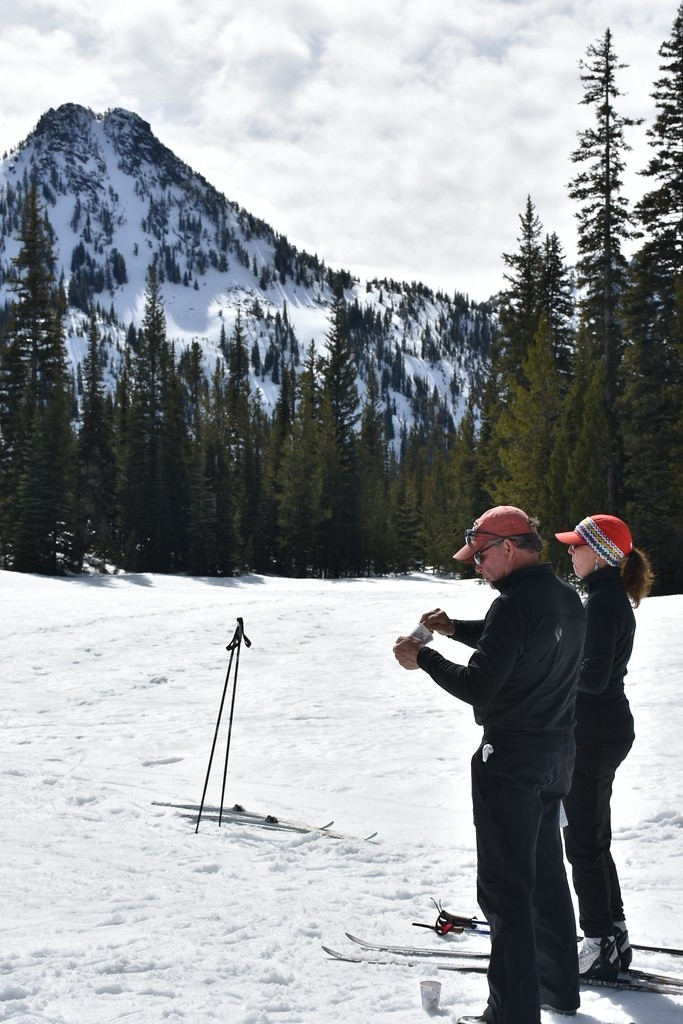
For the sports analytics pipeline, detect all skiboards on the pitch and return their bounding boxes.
[149,799,378,843]
[320,930,683,996]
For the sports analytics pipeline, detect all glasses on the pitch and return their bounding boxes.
[474,538,517,564]
[572,544,586,549]
[465,529,517,545]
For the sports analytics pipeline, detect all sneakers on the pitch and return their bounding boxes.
[613,921,632,969]
[577,934,621,979]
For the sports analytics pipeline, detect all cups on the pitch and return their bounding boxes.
[406,622,434,645]
[420,981,442,1009]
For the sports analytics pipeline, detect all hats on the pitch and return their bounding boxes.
[453,506,537,563]
[555,515,633,556]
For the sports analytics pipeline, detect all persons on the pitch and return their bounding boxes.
[393,506,587,1024]
[555,515,654,979]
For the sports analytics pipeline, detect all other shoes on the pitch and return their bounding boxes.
[540,1003,576,1016]
[457,1015,487,1024]
